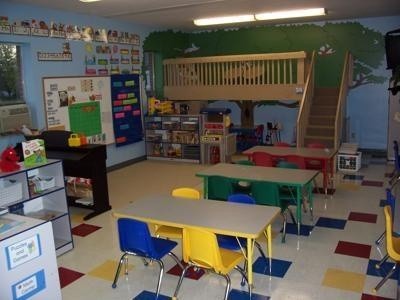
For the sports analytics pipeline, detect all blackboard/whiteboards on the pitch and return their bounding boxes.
[41,76,115,146]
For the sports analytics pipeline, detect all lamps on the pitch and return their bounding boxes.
[193,7,328,28]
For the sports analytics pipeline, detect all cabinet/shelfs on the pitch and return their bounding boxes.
[143,114,208,164]
[0,159,75,300]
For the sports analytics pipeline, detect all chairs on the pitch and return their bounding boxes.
[111,142,337,300]
[370,140,400,292]
